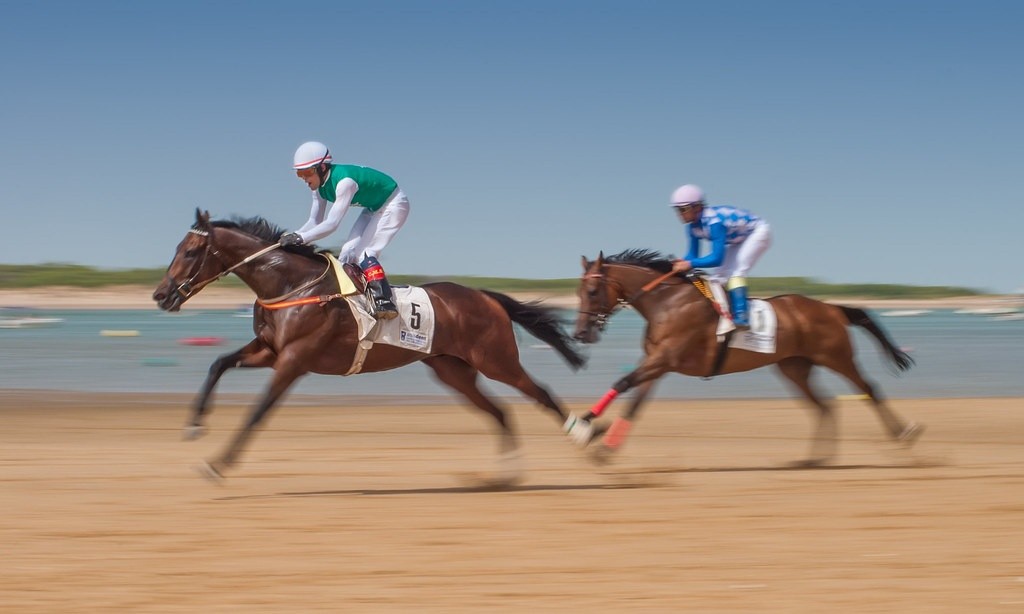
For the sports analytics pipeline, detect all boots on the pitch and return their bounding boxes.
[360,256,399,321]
[725,276,750,333]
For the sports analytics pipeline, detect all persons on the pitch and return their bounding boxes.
[670,184,772,329]
[277,141,411,320]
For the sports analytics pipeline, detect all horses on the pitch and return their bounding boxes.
[152,207,924,479]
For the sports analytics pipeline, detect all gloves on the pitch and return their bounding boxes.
[278,233,303,250]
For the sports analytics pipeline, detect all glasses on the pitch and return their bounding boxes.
[676,206,693,214]
[297,170,317,178]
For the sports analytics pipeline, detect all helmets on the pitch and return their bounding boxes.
[292,141,333,169]
[668,184,706,206]
